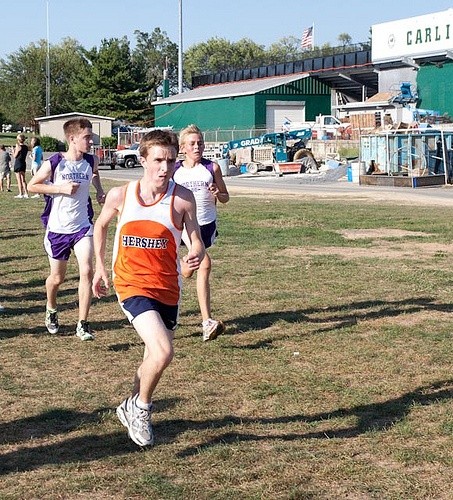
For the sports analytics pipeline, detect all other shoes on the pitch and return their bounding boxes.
[5,189,13,192]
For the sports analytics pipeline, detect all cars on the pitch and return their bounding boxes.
[113,142,143,168]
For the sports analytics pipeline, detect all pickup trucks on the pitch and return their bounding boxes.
[284,115,351,136]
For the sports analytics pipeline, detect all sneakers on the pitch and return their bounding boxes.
[116,393,154,446]
[76,321,94,340]
[202,318,225,342]
[45,305,58,334]
[14,194,29,198]
[31,194,41,198]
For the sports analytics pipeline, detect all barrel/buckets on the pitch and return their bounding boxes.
[351,161,366,184]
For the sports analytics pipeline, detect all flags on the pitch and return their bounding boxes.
[299,26,313,48]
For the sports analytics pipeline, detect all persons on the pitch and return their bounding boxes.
[169,123,229,342]
[28,136,43,199]
[92,129,206,447]
[0,133,28,198]
[27,117,106,340]
